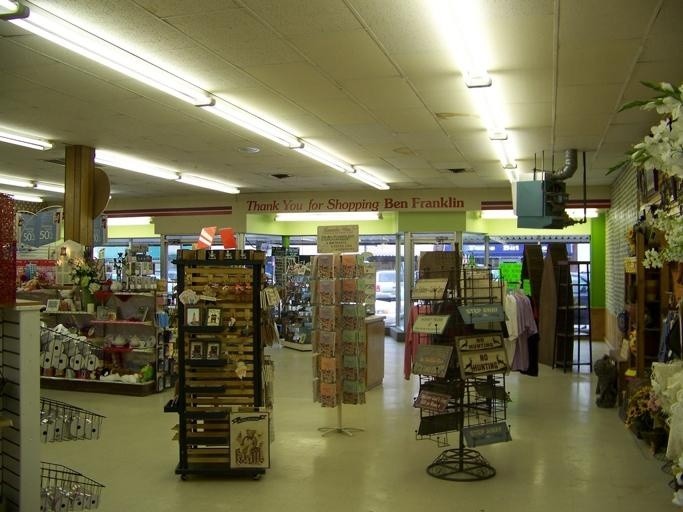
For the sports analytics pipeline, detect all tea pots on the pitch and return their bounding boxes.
[109,333,144,348]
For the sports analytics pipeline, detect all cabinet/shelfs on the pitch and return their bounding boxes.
[412,267,507,484]
[16,283,170,397]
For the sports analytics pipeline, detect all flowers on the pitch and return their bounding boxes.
[603,80,682,269]
[624,384,664,427]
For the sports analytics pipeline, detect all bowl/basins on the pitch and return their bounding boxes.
[60,289,74,298]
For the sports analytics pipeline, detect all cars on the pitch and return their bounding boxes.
[570,271,591,324]
[376,271,396,300]
